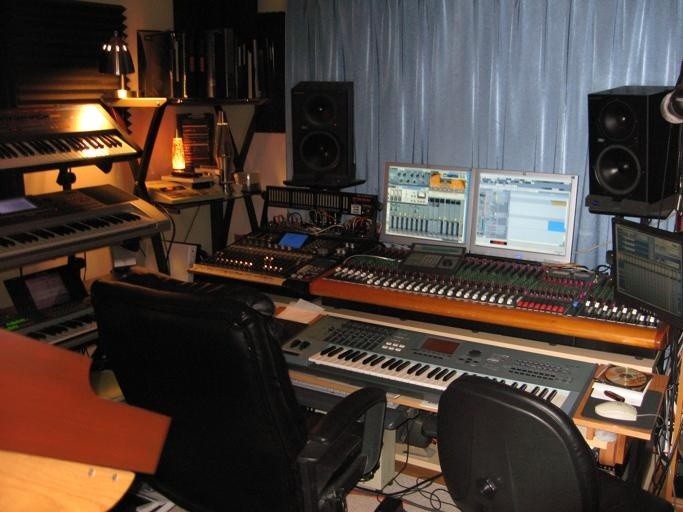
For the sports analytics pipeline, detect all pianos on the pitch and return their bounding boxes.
[0,286,99,351]
[279,313,598,425]
[0,102,145,175]
[0,184,172,271]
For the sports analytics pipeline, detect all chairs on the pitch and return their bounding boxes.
[90,272,387,512]
[436,375,683,512]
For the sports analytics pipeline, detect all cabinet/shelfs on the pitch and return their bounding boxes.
[101,97,262,275]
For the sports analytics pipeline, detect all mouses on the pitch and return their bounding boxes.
[594,401,637,422]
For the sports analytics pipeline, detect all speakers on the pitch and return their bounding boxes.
[588,86,683,220]
[290,81,356,188]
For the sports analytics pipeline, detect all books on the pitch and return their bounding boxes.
[175,112,214,170]
[133,27,266,100]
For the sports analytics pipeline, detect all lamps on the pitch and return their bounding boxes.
[96,30,135,99]
[170,129,193,177]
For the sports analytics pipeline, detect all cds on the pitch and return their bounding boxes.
[604,366,646,387]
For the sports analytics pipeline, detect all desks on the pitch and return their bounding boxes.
[189,241,683,512]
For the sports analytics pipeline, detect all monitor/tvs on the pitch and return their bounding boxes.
[470,168,579,268]
[612,217,683,331]
[381,162,475,254]
